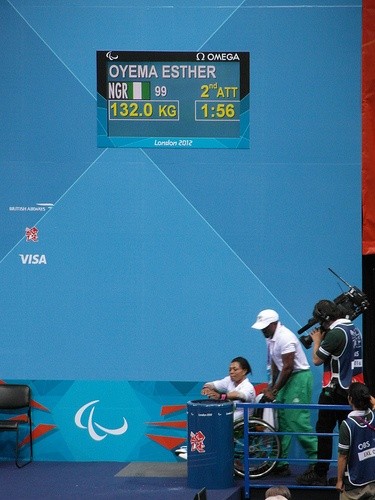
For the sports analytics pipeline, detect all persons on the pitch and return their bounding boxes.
[174,356,256,461]
[335,382,375,500]
[265,485,291,500]
[296,301,366,486]
[251,310,319,486]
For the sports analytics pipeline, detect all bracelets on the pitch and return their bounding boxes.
[202,386,210,389]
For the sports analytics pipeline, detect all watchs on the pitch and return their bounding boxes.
[270,386,279,396]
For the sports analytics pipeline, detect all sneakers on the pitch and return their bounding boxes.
[269,464,291,476]
[299,463,328,485]
[175,445,188,460]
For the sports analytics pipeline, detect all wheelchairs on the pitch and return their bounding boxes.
[232,392,283,480]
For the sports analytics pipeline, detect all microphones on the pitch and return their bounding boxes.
[298,319,317,334]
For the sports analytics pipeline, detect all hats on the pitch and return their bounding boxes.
[251,309,279,330]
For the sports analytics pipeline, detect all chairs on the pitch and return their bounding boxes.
[0,384,34,468]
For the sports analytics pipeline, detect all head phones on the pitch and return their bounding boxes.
[315,303,330,324]
